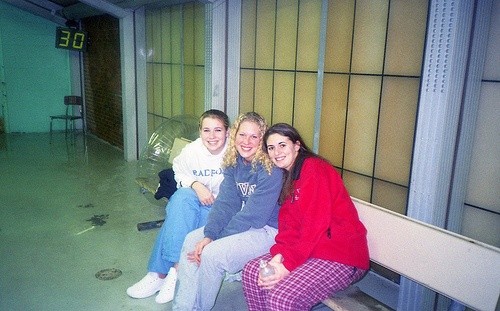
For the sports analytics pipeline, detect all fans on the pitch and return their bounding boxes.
[137,114,201,206]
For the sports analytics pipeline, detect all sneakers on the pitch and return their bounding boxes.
[156,267,177,304]
[126,272,166,298]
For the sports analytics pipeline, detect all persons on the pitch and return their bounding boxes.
[242,123,370,311]
[126,109,231,303]
[171,111,283,311]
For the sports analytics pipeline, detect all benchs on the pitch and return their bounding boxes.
[134,137,500,311]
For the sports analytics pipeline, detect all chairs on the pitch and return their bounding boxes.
[49,96,86,136]
